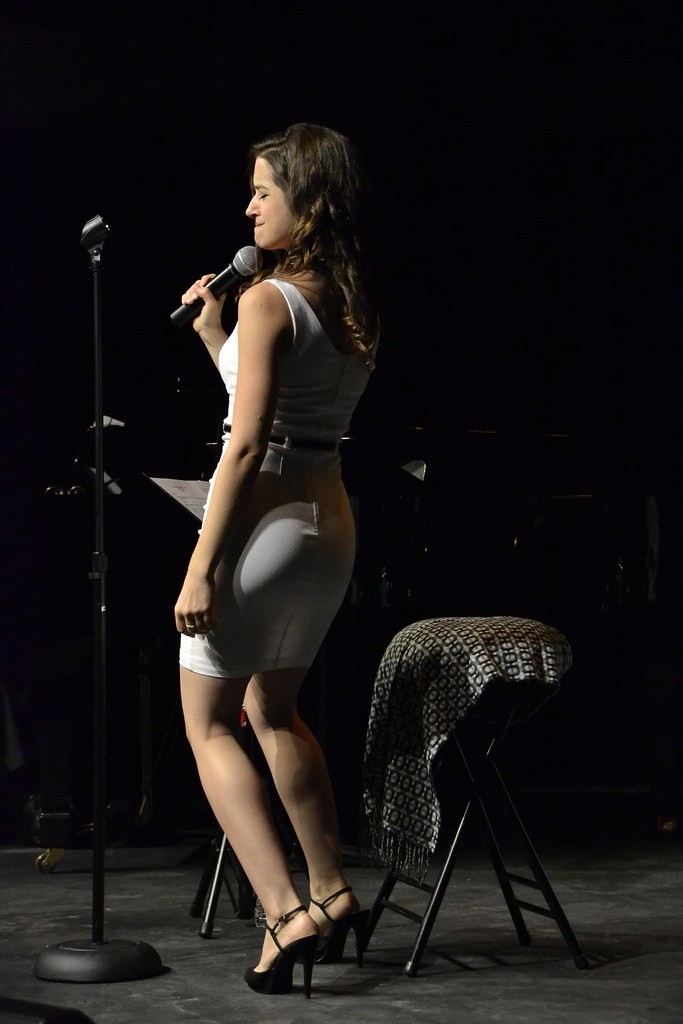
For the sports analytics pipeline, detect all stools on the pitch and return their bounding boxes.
[360,615,590,978]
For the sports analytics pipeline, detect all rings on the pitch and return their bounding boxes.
[186,624,194,630]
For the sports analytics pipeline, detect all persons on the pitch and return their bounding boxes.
[174,123,382,998]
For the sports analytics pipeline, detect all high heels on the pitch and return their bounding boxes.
[295,883,374,972]
[243,905,320,999]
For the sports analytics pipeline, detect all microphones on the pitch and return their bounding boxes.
[170,246,264,327]
